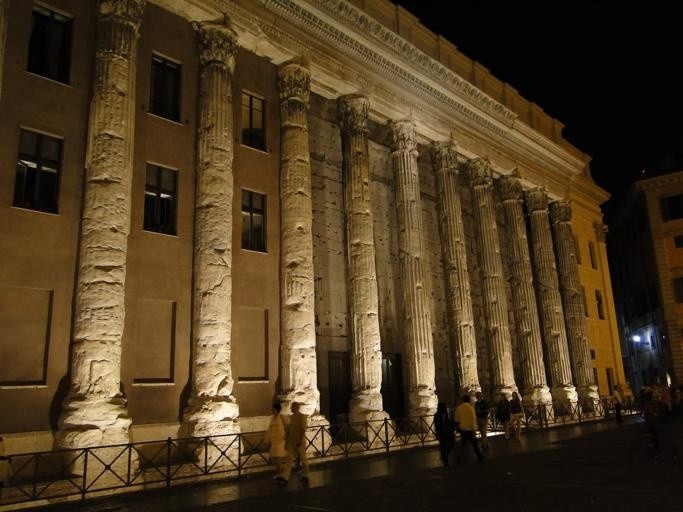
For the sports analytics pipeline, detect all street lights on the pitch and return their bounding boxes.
[624,325,640,416]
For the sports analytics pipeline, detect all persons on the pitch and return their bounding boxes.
[434,402,460,471]
[279,402,309,481]
[613,385,624,424]
[452,395,491,472]
[266,403,292,483]
[474,392,489,436]
[496,394,512,440]
[510,391,525,439]
